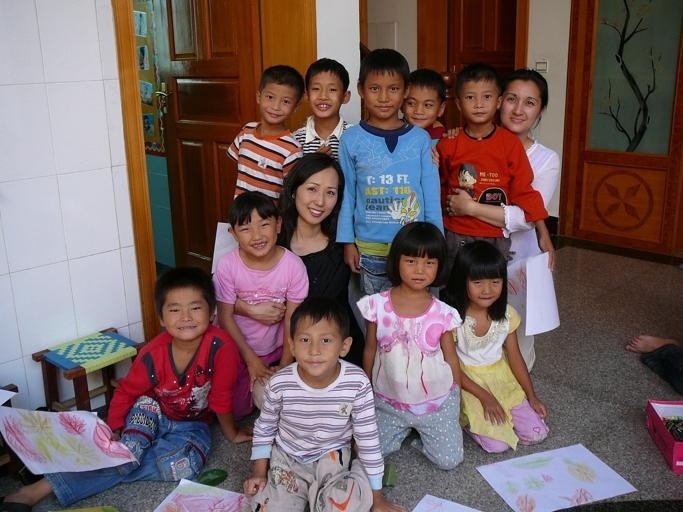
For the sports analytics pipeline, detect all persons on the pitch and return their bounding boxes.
[624,335,683,396]
[0,266,255,512]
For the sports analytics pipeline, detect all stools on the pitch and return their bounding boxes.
[0,383,23,478]
[32,327,145,414]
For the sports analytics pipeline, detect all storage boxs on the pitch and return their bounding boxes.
[646,399,683,474]
